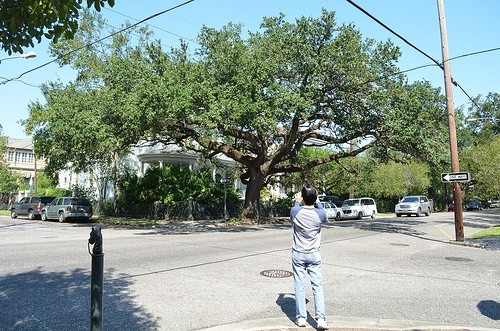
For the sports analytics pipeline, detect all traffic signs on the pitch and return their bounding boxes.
[441,172,472,183]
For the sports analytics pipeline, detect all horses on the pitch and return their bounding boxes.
[88,226,102,254]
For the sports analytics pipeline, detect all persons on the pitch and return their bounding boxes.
[289,185,329,330]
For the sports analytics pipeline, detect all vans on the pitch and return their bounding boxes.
[339,197,377,220]
[319,196,341,206]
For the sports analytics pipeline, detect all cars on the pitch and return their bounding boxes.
[490,202,500,208]
[448,200,483,212]
[320,202,343,221]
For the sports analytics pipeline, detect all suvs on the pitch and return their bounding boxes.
[39,197,92,223]
[9,196,57,220]
[394,196,431,217]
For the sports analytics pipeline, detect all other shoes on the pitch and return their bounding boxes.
[315,318,328,329]
[295,317,307,327]
